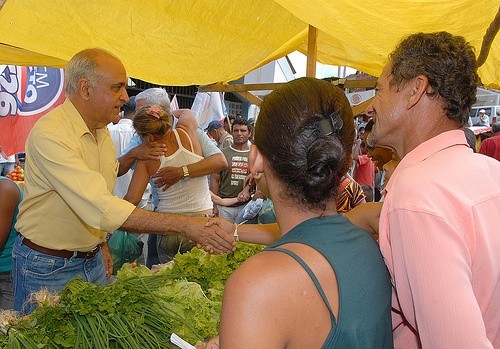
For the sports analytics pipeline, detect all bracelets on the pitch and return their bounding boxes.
[233,220,248,243]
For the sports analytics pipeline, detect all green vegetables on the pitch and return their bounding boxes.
[9,276,149,349]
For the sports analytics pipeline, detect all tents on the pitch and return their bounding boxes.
[1,0,499,121]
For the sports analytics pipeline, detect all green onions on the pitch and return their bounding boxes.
[0,274,204,349]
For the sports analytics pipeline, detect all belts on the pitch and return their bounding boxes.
[19,233,102,259]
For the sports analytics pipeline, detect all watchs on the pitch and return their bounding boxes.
[181,165,191,182]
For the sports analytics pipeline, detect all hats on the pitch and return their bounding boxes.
[479,109,485,113]
[204,120,221,132]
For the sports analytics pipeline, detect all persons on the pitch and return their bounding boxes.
[336,176,366,215]
[203,115,267,225]
[196,149,401,255]
[352,114,394,203]
[195,76,394,349]
[115,87,228,271]
[366,31,500,349]
[11,48,237,320]
[464,108,500,162]
[0,175,25,312]
[0,147,20,178]
[120,103,214,264]
[107,96,155,267]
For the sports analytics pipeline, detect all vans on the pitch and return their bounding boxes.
[471,105,500,126]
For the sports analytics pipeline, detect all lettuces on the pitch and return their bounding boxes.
[114,242,270,344]
